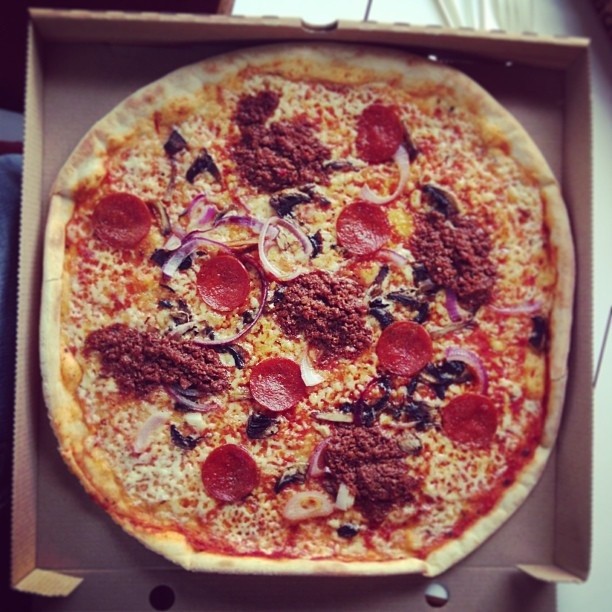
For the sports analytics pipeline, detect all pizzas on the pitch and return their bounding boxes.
[38,37,578,579]
